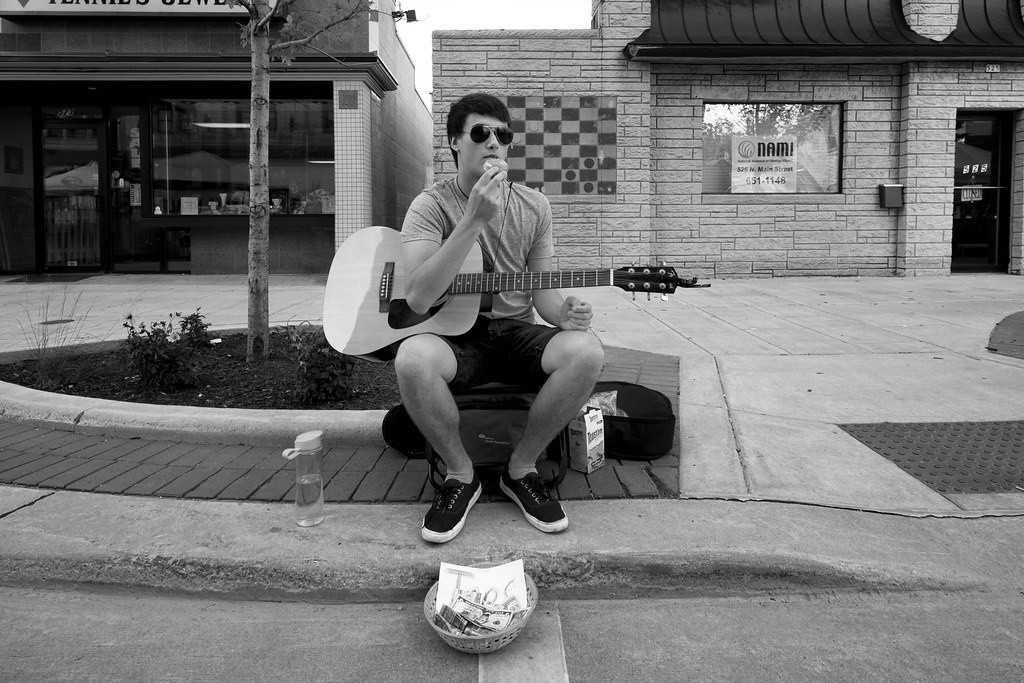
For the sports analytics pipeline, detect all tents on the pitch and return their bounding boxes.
[44,160,99,196]
[153,150,290,214]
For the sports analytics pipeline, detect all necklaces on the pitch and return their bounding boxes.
[456,175,469,198]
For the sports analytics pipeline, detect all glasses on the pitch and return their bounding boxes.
[461,122,515,147]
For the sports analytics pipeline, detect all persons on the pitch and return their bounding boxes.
[395,93,604,542]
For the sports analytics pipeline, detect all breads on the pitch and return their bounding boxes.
[483,158,508,177]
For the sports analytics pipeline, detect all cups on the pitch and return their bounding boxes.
[282,431,324,527]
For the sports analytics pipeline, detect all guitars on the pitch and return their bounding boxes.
[323,225,714,364]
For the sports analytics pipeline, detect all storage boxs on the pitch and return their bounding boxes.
[879,183,904,208]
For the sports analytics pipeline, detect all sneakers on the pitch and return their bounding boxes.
[497,465,570,533]
[419,467,482,544]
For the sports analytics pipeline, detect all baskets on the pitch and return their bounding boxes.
[423,561,539,653]
